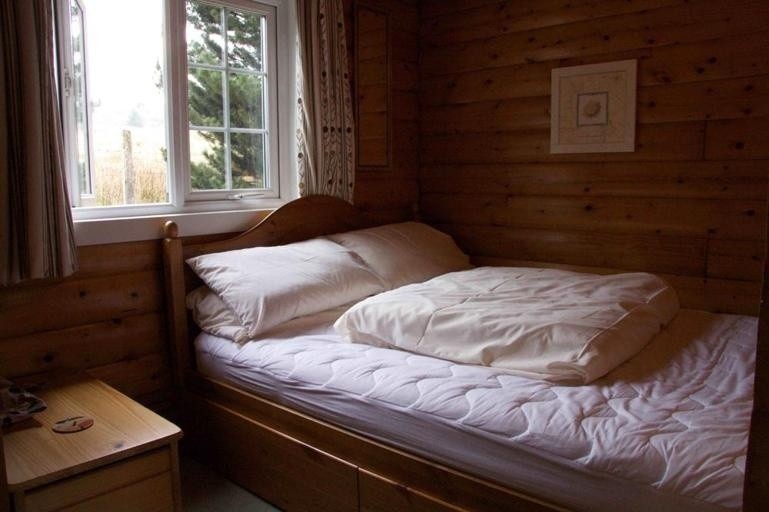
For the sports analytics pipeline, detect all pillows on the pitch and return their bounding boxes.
[332,220,475,289]
[185,236,385,338]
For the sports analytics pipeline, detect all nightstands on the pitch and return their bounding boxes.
[0,376,182,512]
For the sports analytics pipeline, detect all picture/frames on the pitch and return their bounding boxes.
[550,59,638,155]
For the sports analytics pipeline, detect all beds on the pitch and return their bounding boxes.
[161,193,759,512]
